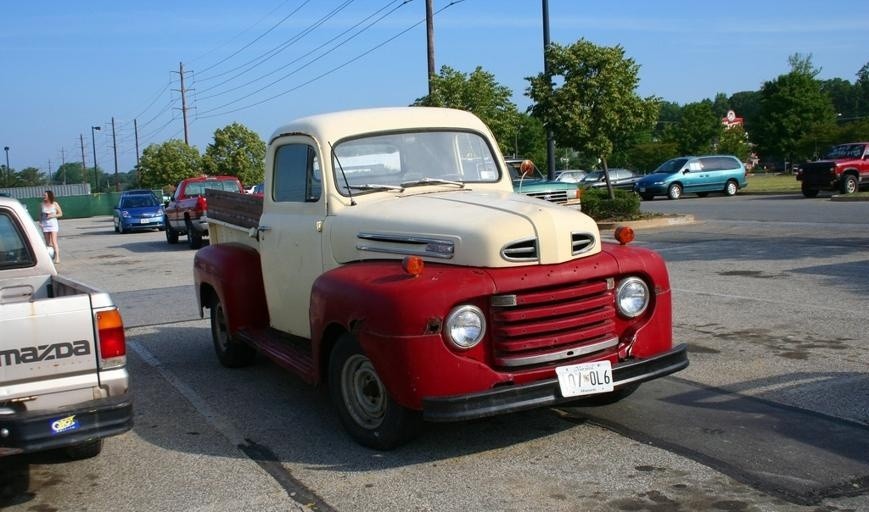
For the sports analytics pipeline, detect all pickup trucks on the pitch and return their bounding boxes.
[0,195,136,460]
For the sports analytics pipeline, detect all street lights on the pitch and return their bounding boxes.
[4,145,11,187]
[91,126,103,192]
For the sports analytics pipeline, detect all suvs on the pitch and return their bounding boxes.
[796,141,869,199]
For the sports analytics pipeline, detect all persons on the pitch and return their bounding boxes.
[37,189,63,264]
